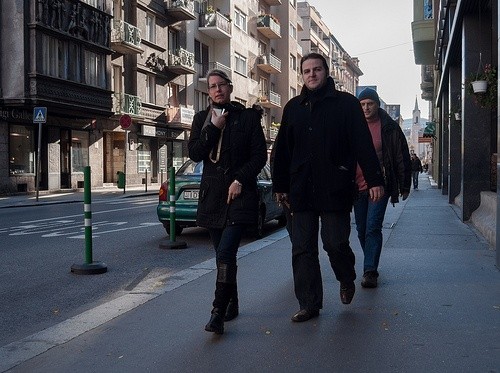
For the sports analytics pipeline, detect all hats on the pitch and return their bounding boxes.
[358,88,380,105]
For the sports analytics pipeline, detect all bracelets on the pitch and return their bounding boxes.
[234,180,243,185]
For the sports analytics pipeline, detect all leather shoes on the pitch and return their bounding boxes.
[292,309,320,322]
[339,280,355,305]
[361,277,377,287]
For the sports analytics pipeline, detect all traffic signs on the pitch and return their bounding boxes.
[33,107,47,123]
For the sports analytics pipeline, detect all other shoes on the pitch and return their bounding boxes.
[416,187,418,190]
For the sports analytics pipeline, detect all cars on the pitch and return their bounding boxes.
[157,159,286,237]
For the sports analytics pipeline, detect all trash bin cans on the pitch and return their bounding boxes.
[117,171,124,188]
[142,178,146,184]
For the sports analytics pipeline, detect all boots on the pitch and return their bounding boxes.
[205,263,238,335]
[211,266,238,320]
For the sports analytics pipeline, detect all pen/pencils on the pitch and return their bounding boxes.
[222,108,225,115]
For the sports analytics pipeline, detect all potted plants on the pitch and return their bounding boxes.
[465,64,498,111]
[448,105,463,121]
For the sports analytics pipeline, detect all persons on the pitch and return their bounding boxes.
[267,52,385,322]
[410,154,424,190]
[420,162,428,174]
[189,69,269,337]
[344,86,412,289]
